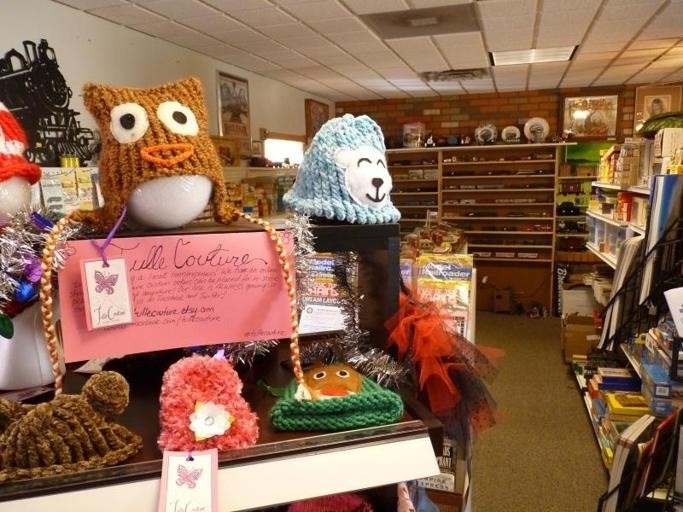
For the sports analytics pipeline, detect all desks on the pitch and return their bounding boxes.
[0,344,441,512]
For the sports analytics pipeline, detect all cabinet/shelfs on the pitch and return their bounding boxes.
[218,161,300,230]
[583,181,683,510]
[387,148,440,242]
[553,144,613,266]
[441,143,553,318]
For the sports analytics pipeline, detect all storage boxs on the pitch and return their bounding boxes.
[561,317,605,365]
[561,283,601,320]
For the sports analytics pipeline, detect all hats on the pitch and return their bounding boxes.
[283,113,402,224]
[269,359,405,433]
[0,102,41,187]
[72,76,239,230]
[0,370,144,485]
[156,355,260,454]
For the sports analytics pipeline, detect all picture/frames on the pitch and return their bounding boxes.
[215,68,251,144]
[555,86,682,144]
